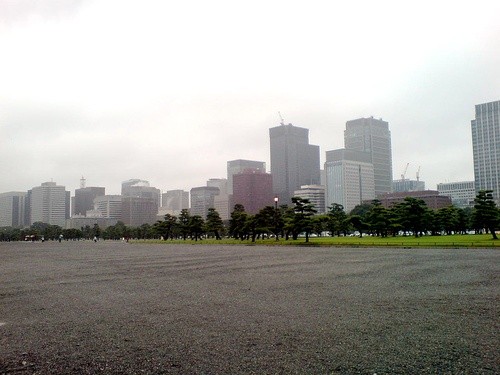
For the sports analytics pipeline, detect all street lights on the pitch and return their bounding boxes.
[274,192,279,242]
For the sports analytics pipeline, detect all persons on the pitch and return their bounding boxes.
[21,232,127,243]
